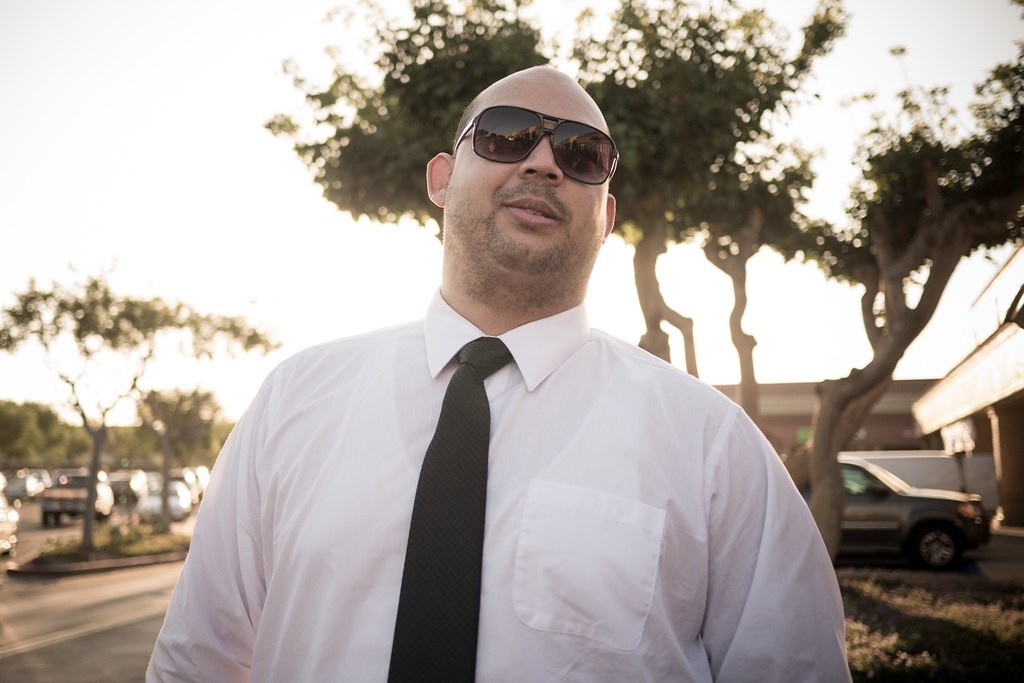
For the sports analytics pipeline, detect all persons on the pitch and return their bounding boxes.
[146,66,850,682]
[484,140,500,161]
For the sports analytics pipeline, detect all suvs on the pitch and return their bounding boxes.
[834,453,993,574]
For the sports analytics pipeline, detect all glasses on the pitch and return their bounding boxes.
[451,105,619,185]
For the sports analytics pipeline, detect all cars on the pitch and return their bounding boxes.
[0,467,210,559]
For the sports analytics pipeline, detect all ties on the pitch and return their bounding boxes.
[388,335,513,683]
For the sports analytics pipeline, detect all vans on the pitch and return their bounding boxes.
[836,445,1000,519]
